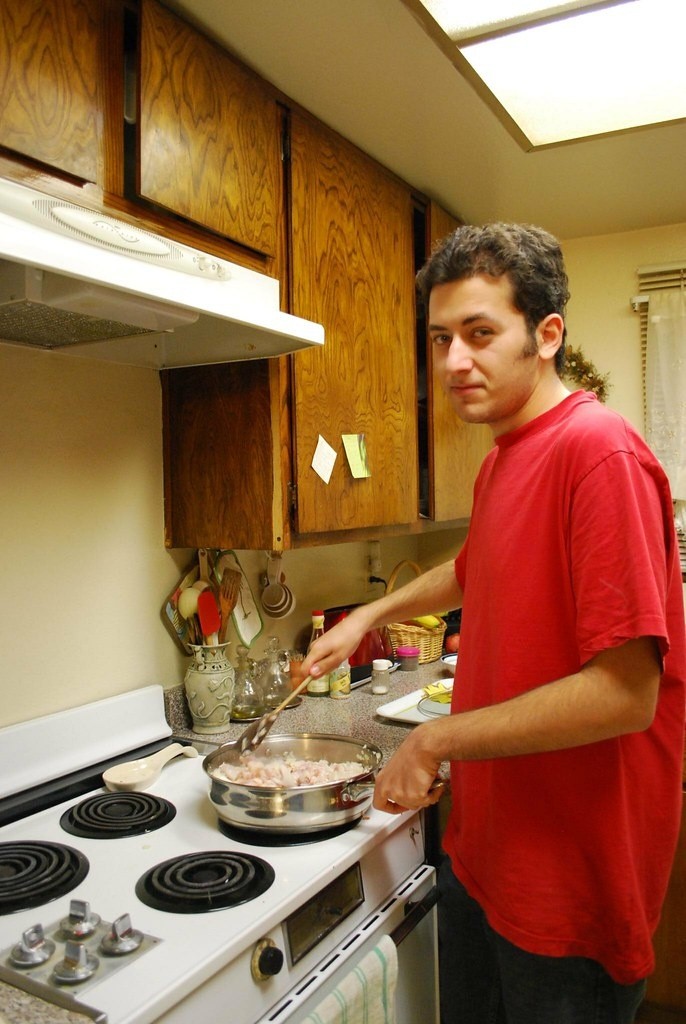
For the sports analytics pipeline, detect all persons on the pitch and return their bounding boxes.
[298,220,682,1024]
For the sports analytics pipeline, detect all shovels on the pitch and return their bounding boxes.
[234,676,316,755]
[218,569,243,645]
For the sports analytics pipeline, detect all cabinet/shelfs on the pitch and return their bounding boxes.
[141,812,440,1024]
[160,98,495,556]
[1,1,286,276]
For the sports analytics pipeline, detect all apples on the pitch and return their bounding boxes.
[445,632,460,653]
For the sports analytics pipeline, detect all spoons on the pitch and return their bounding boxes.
[261,555,296,620]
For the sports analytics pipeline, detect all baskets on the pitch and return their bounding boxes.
[382,560,448,664]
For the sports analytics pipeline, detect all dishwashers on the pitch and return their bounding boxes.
[252,864,442,1024]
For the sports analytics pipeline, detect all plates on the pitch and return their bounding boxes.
[376,678,454,725]
[230,708,274,722]
[266,696,304,710]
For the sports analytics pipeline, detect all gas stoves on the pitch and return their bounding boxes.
[1,683,430,1024]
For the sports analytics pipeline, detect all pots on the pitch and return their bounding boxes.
[202,732,384,835]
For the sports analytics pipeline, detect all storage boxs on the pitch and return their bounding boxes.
[384,560,447,665]
[184,639,237,735]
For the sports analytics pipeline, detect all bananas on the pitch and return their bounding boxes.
[409,612,448,628]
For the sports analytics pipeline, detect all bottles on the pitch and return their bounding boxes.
[329,612,351,699]
[230,645,264,719]
[258,636,293,707]
[306,610,329,697]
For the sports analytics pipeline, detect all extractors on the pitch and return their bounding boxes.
[0,174,326,372]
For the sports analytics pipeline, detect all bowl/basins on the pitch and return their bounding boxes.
[441,652,458,675]
